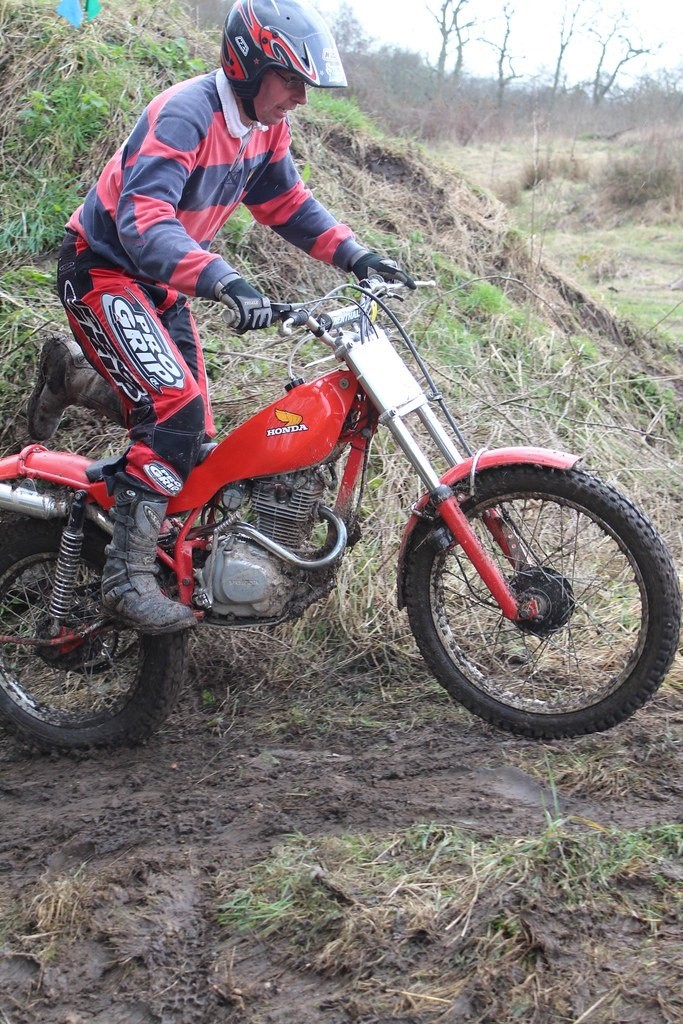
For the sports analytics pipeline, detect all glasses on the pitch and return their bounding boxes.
[271,67,307,89]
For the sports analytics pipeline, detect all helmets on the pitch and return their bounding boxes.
[220,0,349,99]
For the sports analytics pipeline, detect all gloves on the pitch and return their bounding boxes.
[352,253,417,289]
[219,278,272,334]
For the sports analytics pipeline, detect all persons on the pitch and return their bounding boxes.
[28,0,416,632]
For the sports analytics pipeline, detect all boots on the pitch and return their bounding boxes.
[103,483,198,636]
[25,338,128,442]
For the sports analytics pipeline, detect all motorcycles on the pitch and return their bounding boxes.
[0,274,682,752]
